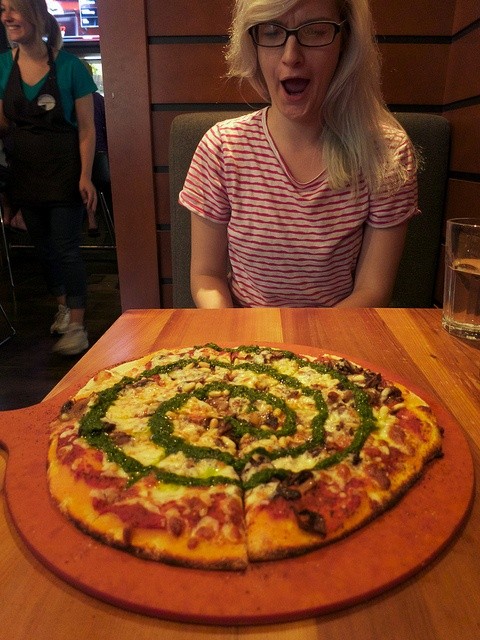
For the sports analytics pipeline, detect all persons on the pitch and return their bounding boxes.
[0,0,95,355]
[177,1,420,308]
[80,57,111,187]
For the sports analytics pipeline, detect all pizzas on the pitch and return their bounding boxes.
[46,342,441,570]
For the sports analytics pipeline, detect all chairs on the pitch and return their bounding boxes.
[170,112,452,309]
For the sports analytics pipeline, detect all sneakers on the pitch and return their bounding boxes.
[50,304,71,335]
[53,322,89,355]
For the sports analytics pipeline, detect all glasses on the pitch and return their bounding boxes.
[248,17,348,48]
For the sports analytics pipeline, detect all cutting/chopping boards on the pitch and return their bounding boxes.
[0,342,478,622]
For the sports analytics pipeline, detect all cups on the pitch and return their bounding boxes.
[443,217,480,342]
